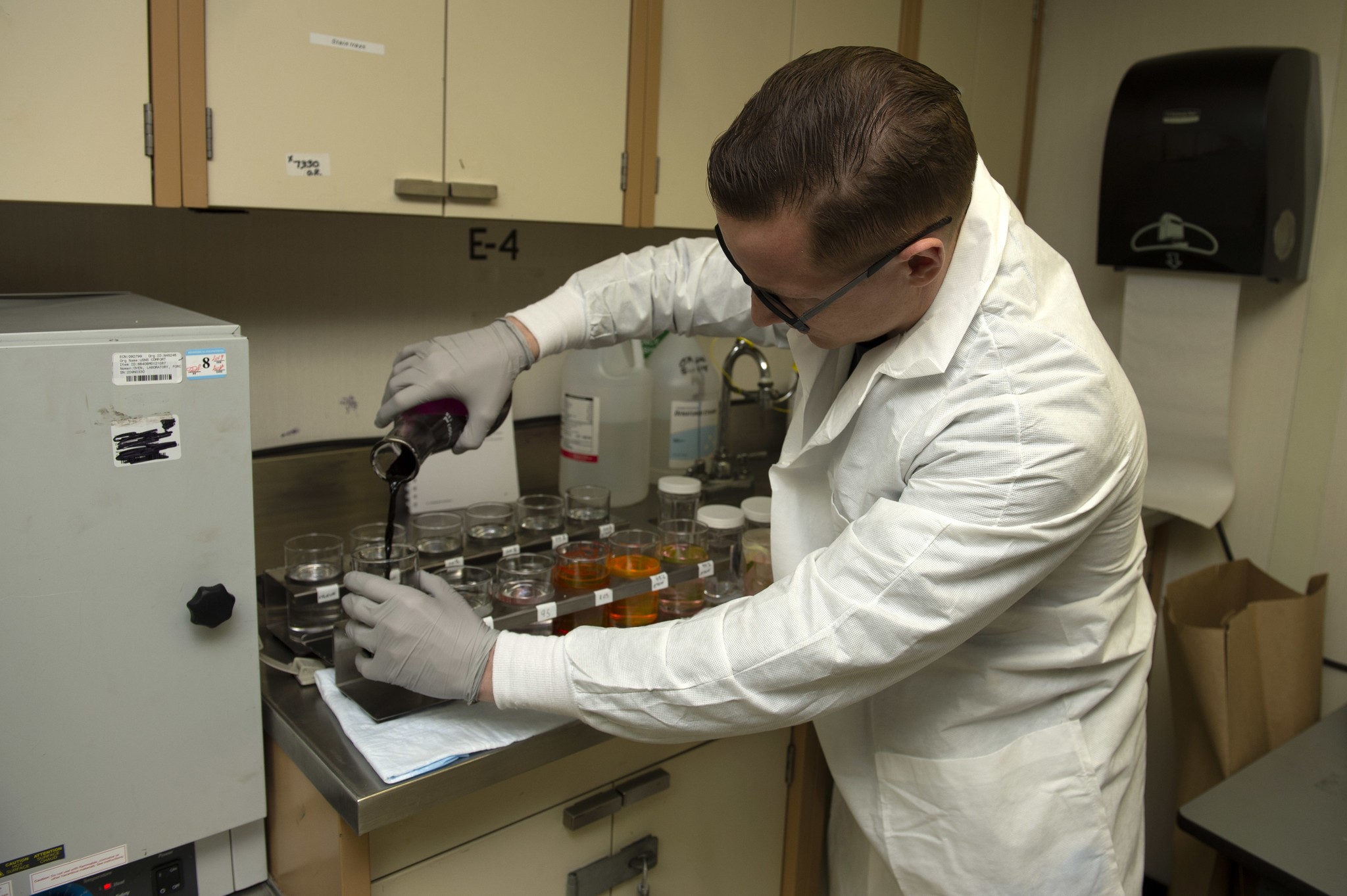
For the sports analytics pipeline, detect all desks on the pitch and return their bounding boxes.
[1176,704,1347,896]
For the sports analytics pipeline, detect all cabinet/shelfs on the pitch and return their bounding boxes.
[0,0,1048,232]
[262,723,833,896]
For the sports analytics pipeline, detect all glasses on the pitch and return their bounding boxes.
[714,214,951,334]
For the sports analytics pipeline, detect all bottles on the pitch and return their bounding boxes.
[647,332,721,482]
[559,337,652,508]
[370,390,513,484]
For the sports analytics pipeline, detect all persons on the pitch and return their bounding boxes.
[342,45,1157,896]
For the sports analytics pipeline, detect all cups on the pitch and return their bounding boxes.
[566,485,611,528]
[658,519,709,614]
[494,552,557,636]
[517,494,565,536]
[556,541,611,633]
[741,497,774,596]
[351,543,420,590]
[283,534,345,643]
[349,523,408,571]
[657,476,702,544]
[609,529,662,628]
[696,504,744,604]
[433,565,493,611]
[464,501,519,546]
[410,511,466,573]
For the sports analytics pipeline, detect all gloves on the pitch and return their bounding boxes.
[374,318,535,455]
[343,569,500,706]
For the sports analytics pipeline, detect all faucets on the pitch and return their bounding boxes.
[685,341,776,490]
